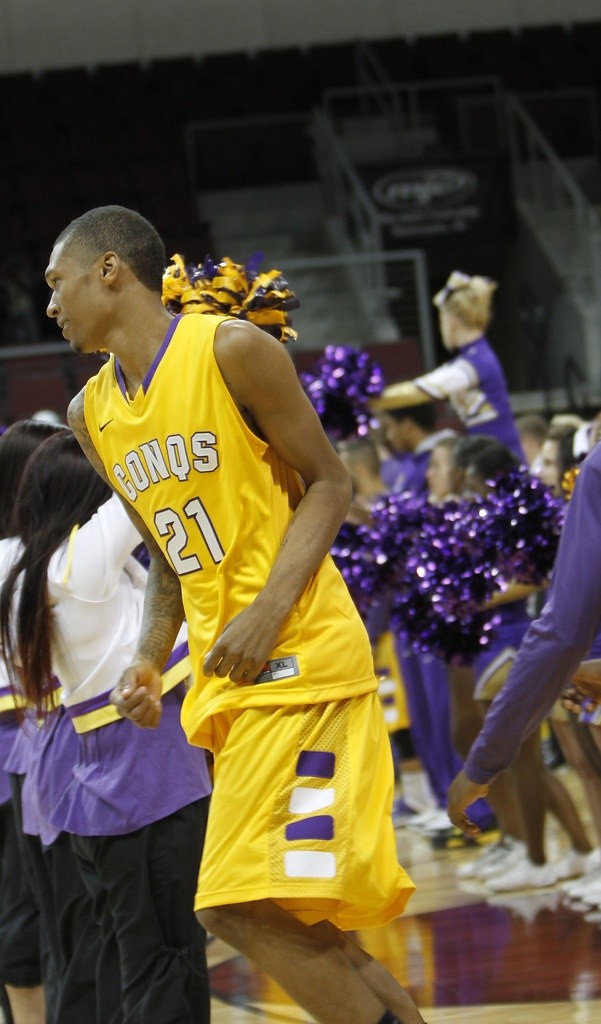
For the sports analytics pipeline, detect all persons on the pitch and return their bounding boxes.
[339,403,601,903]
[364,272,525,465]
[0,420,213,1024]
[44,204,430,1024]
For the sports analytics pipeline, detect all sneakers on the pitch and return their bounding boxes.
[567,874,601,904]
[482,859,558,890]
[555,846,600,880]
[453,831,532,882]
